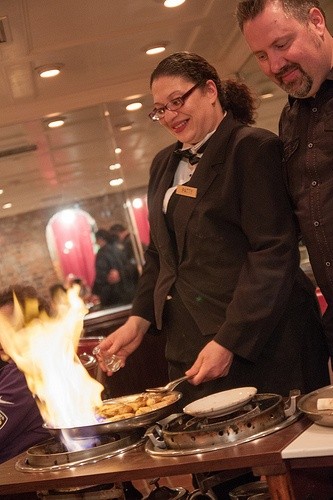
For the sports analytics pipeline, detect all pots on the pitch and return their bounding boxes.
[42,377,190,436]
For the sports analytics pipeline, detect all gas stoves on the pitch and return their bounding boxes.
[0,389,314,500]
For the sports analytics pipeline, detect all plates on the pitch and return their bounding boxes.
[182,386,257,418]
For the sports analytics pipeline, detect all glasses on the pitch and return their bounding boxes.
[148,79,203,121]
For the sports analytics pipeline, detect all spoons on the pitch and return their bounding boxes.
[145,374,187,391]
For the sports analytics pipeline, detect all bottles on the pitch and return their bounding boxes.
[92,346,122,372]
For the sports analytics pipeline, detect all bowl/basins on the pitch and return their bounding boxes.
[296,384,333,426]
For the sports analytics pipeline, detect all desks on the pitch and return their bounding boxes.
[0,396,333,500]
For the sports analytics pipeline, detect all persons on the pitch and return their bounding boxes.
[96,51,331,413]
[0,283,53,465]
[108,224,148,303]
[92,228,131,308]
[233,0,333,373]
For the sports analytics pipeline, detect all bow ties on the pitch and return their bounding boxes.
[172,135,212,166]
